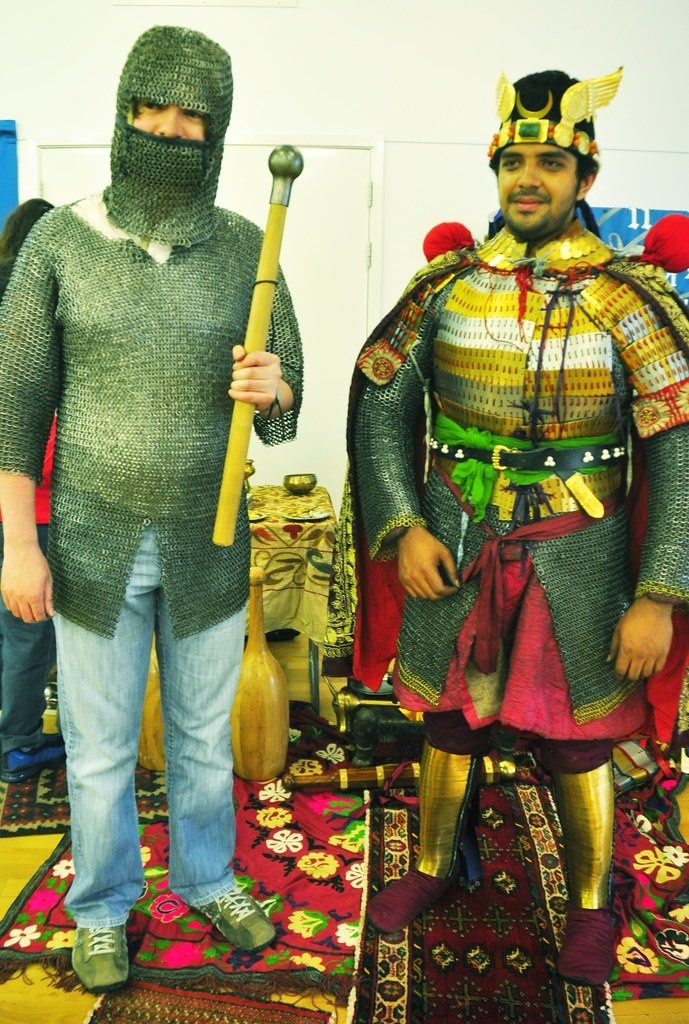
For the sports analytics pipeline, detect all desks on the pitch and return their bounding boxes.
[247,482,336,713]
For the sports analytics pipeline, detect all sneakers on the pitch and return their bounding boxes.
[0,733,66,782]
[71,919,129,992]
[186,882,277,953]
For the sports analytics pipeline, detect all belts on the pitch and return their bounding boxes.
[428,437,627,518]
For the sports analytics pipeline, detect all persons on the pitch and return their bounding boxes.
[0,26,304,993]
[0,199,56,781]
[346,68,688,985]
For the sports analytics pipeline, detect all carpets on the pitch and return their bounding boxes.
[0,700,689,1024]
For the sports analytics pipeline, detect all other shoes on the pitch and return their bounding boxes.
[366,869,450,933]
[558,901,616,988]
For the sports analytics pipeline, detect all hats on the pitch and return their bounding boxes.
[488,67,624,171]
